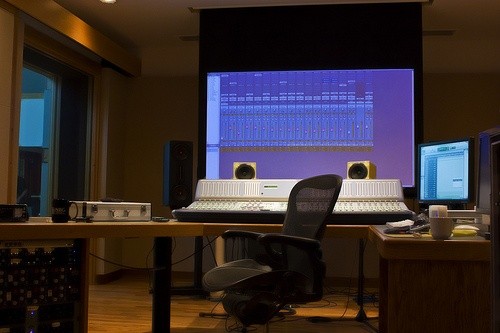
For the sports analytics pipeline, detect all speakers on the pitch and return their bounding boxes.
[346,160,376,179]
[164,142,191,208]
[232,161,256,179]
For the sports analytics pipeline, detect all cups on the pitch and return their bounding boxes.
[51,198,78,223]
[429,217,453,240]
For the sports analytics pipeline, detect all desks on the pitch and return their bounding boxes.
[170,222,369,303]
[370,224,493,333]
[0,216,204,333]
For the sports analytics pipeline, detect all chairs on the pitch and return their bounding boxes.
[220,174,342,301]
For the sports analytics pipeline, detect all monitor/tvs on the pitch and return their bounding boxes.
[419,137,478,203]
